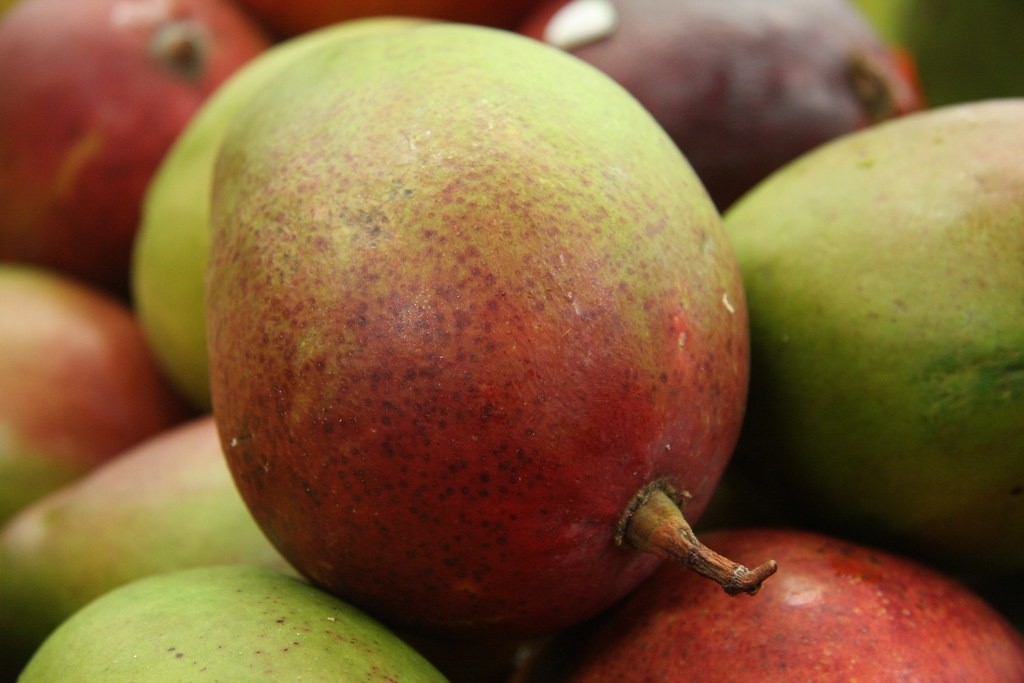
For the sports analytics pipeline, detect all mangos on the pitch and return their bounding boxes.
[0,0,1024,683]
